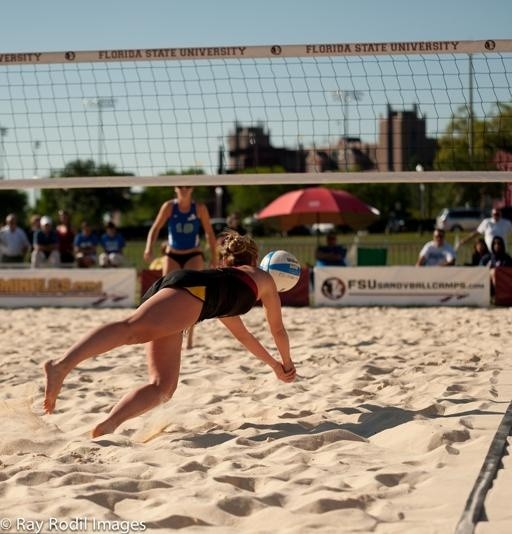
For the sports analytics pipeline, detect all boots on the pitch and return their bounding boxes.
[307,222,334,235]
[434,207,492,232]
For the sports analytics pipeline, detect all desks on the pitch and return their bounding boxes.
[83,96,119,173]
[331,87,361,171]
[32,137,41,200]
[0,126,9,182]
[413,164,427,233]
[213,185,223,231]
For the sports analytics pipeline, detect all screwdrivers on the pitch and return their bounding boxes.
[259,250,300,291]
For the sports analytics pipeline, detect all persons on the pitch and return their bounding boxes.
[41,230,298,442]
[144,185,219,350]
[1,194,510,309]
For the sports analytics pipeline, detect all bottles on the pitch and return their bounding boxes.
[39,215,53,226]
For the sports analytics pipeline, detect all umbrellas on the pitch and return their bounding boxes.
[253,184,377,266]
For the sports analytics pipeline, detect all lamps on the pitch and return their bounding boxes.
[354,245,388,264]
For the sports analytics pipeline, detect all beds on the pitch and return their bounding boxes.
[253,184,377,266]
